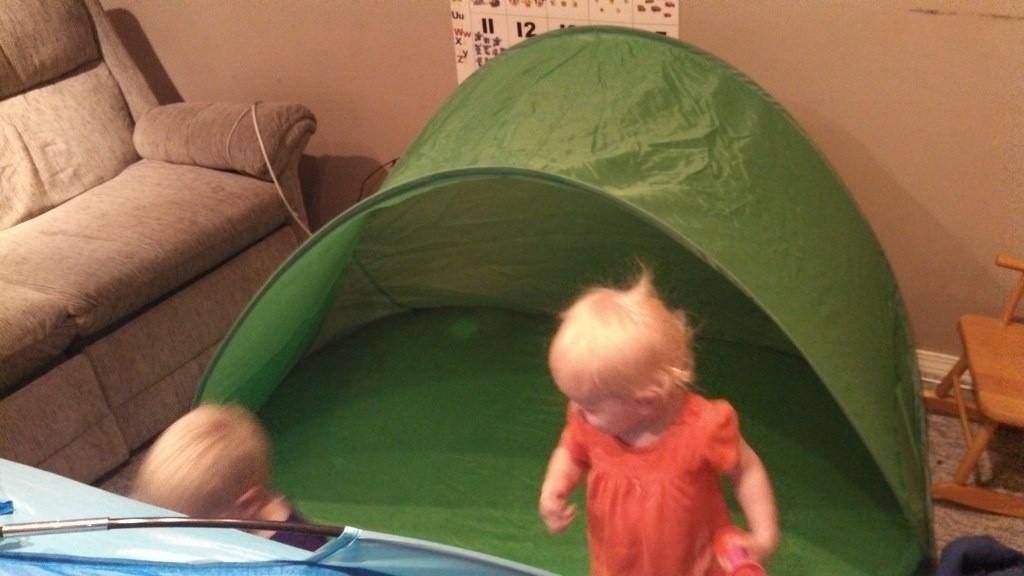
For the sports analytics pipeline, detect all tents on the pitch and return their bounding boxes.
[0,459,565,576]
[190,25,941,576]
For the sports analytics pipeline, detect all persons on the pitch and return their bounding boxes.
[537,266,778,576]
[125,397,329,552]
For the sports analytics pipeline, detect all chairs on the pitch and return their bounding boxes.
[921,255,1024,518]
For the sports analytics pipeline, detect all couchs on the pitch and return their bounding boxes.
[1,2,320,486]
